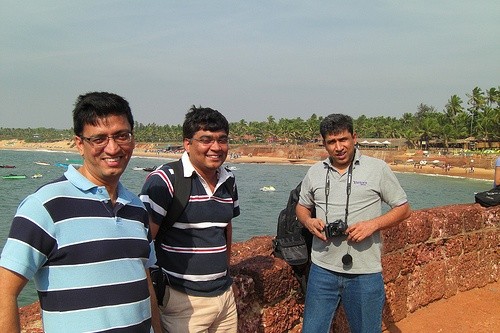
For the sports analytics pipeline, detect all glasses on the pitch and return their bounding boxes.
[192,138,228,144]
[79,131,133,147]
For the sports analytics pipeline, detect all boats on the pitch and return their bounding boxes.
[132,165,157,172]
[54,158,83,168]
[0,173,27,179]
[0,164,16,168]
[259,185,276,192]
[33,160,51,166]
[31,172,43,179]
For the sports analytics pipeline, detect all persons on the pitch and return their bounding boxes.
[493,157,500,187]
[295,114,411,333]
[0,92,163,333]
[138,104,240,333]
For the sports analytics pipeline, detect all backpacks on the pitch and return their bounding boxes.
[272,180,317,299]
[149,161,235,306]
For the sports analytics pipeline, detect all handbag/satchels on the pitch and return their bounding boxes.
[475,187,500,207]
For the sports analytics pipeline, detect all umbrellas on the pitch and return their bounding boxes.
[359,141,370,148]
[371,140,381,148]
[382,140,391,149]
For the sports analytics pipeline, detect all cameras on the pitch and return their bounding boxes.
[325,219,348,238]
